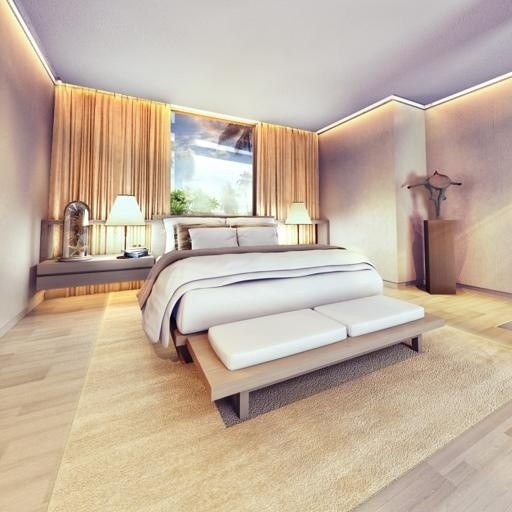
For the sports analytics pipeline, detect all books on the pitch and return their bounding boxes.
[121,247,149,258]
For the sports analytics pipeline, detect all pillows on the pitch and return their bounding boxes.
[163,217,280,257]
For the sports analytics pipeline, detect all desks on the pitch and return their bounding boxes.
[35,250,155,291]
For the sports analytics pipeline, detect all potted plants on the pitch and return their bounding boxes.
[169,188,187,215]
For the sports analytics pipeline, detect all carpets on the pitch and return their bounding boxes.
[46,290,512,512]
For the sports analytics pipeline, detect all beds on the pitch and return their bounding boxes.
[136,214,446,419]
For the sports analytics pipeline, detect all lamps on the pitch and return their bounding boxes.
[103,194,147,259]
[284,202,313,245]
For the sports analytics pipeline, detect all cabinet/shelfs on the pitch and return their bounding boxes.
[423,219,457,294]
